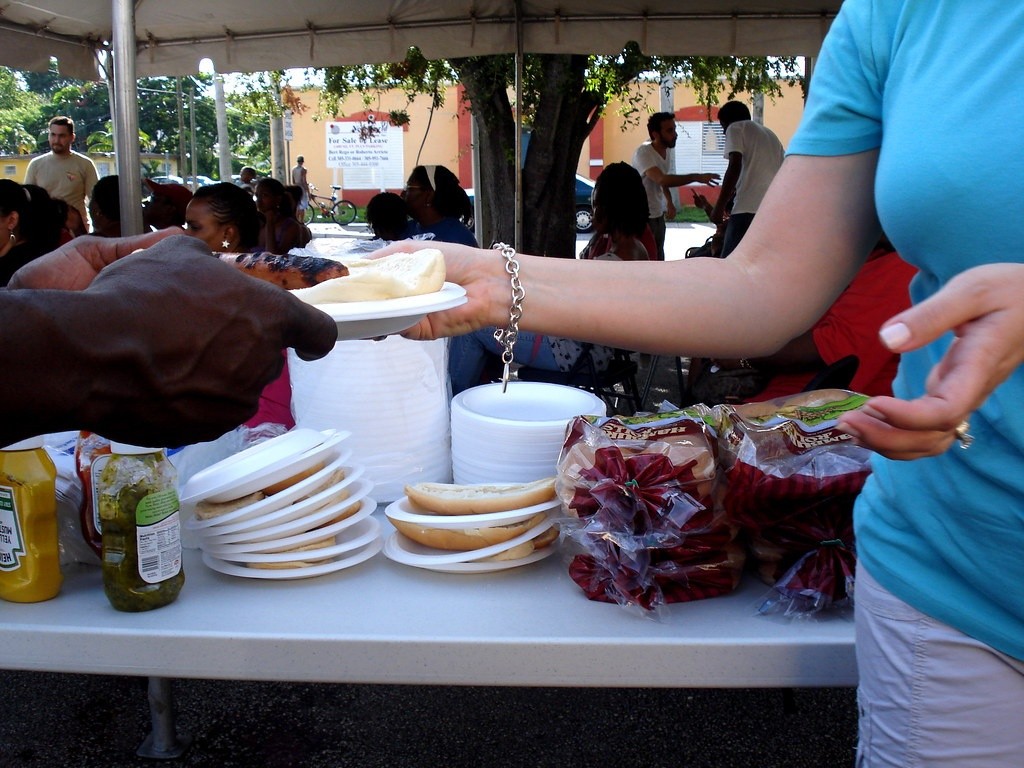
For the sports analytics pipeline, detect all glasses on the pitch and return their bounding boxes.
[404,181,430,192]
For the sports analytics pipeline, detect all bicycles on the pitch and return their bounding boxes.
[303,183,357,226]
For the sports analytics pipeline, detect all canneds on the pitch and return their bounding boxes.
[0,434,63,604]
[99,440,186,612]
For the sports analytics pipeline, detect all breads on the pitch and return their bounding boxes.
[385,476,562,561]
[196,459,362,568]
[286,248,446,303]
[563,390,865,601]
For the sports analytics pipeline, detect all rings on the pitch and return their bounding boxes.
[956,421,975,449]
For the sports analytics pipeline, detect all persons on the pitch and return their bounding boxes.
[0,102,919,462]
[348,1,1024,767]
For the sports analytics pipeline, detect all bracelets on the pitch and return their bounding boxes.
[490,243,525,395]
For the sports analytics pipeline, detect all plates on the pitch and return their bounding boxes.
[183,278,606,580]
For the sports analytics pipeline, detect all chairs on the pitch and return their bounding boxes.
[517,235,654,415]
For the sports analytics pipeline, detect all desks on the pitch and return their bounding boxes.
[0,502,855,761]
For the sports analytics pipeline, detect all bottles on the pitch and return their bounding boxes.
[101,440,185,612]
[76,429,110,561]
[0,434,64,603]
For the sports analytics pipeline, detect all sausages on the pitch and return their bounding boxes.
[211,250,350,289]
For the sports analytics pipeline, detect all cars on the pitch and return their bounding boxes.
[187,175,220,185]
[459,173,596,234]
[150,176,202,194]
